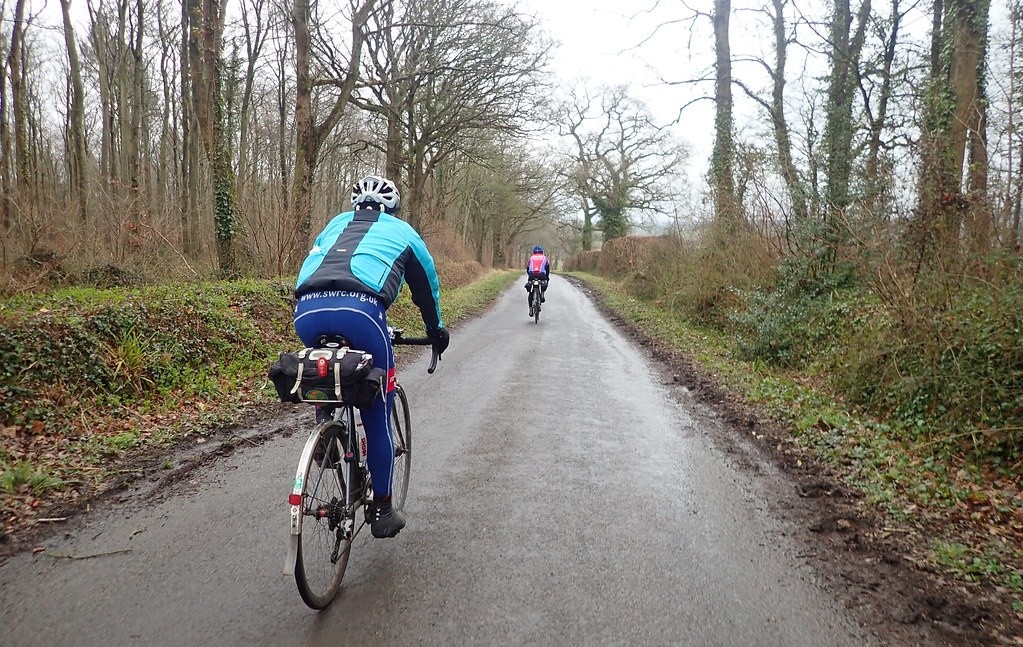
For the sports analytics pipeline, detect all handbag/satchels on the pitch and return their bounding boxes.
[268,347,387,408]
[525,280,547,292]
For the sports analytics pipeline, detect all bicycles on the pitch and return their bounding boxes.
[283,329,442,610]
[528,278,545,324]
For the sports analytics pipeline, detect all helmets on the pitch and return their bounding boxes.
[533,245,543,252]
[351,176,401,208]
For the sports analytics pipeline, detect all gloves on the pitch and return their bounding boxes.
[426,328,449,354]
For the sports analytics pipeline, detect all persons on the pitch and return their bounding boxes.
[293,175,450,538]
[523,246,549,316]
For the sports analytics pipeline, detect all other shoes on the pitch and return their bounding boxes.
[314,439,341,464]
[541,293,545,302]
[529,307,533,316]
[371,506,405,539]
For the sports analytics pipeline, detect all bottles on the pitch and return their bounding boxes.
[355,408,368,461]
